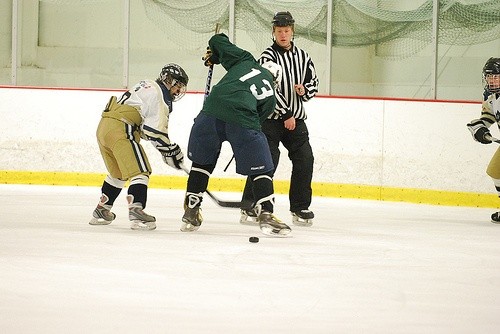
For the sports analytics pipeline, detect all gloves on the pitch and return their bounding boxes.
[474,127,492,144]
[161,148,184,170]
[204,49,214,66]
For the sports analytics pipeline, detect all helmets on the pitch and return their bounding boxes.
[262,61,282,88]
[159,62,189,102]
[271,11,295,27]
[481,56,500,93]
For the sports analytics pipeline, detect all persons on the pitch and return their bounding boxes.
[241,12,318,220]
[92,62,188,222]
[467,57,500,222]
[182,33,291,235]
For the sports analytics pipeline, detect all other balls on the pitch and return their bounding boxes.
[249,237,259,243]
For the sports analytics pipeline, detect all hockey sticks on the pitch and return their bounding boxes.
[181,166,241,208]
[203,24,221,107]
[483,132,500,144]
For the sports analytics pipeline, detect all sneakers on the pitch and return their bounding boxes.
[290,209,314,225]
[257,211,292,238]
[180,191,203,232]
[239,202,259,224]
[490,211,500,224]
[128,202,157,230]
[89,203,116,225]
